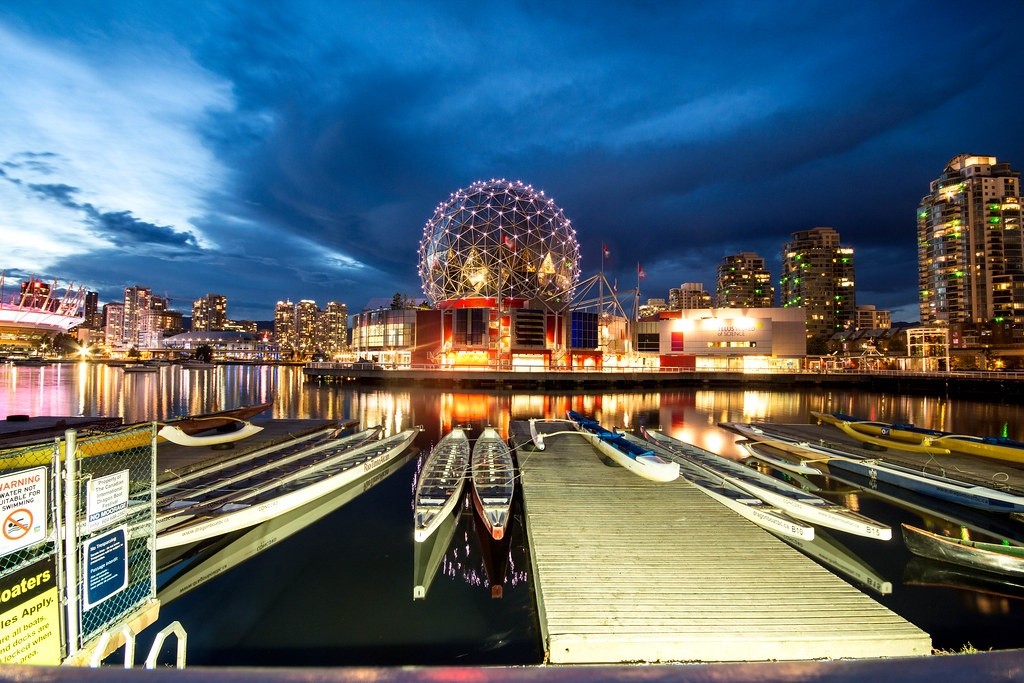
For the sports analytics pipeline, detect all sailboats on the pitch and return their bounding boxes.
[121,330,161,373]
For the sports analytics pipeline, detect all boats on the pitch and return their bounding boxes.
[731,424,1024,515]
[470,426,515,540]
[156,447,420,606]
[611,426,816,542]
[900,557,1024,601]
[142,356,173,366]
[216,361,254,365]
[145,424,421,552]
[1,401,276,471]
[901,522,1024,577]
[107,363,126,367]
[737,455,822,492]
[740,442,823,476]
[409,423,469,543]
[95,425,388,543]
[566,409,682,482]
[810,409,1024,462]
[412,488,467,601]
[0,356,52,366]
[181,359,215,368]
[636,423,892,541]
[470,503,513,600]
[758,519,894,595]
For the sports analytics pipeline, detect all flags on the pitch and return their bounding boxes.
[501,230,514,249]
[636,266,647,280]
[603,243,610,259]
